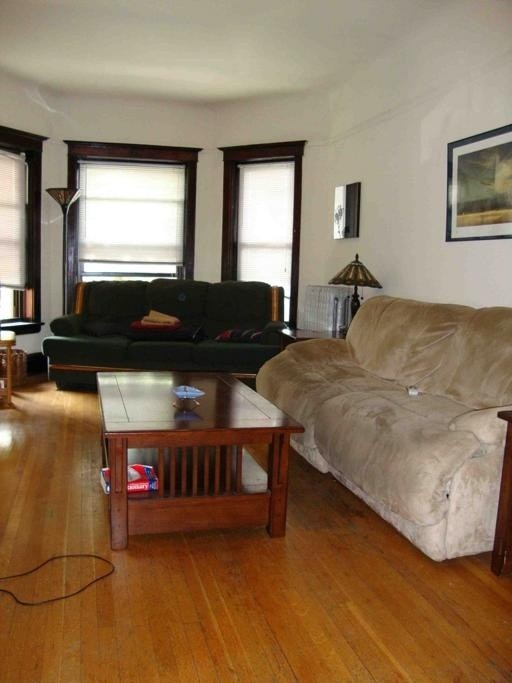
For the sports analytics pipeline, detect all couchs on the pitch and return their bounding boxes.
[42,279,283,390]
[255,295,512,562]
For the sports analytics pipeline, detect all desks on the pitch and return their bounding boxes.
[0,330,15,404]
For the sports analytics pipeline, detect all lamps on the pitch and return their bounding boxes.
[45,188,80,315]
[328,253,383,336]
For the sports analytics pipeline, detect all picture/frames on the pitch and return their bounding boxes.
[446,123,512,241]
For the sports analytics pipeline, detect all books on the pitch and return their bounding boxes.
[98,462,161,495]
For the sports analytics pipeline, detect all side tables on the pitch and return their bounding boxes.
[277,329,346,352]
